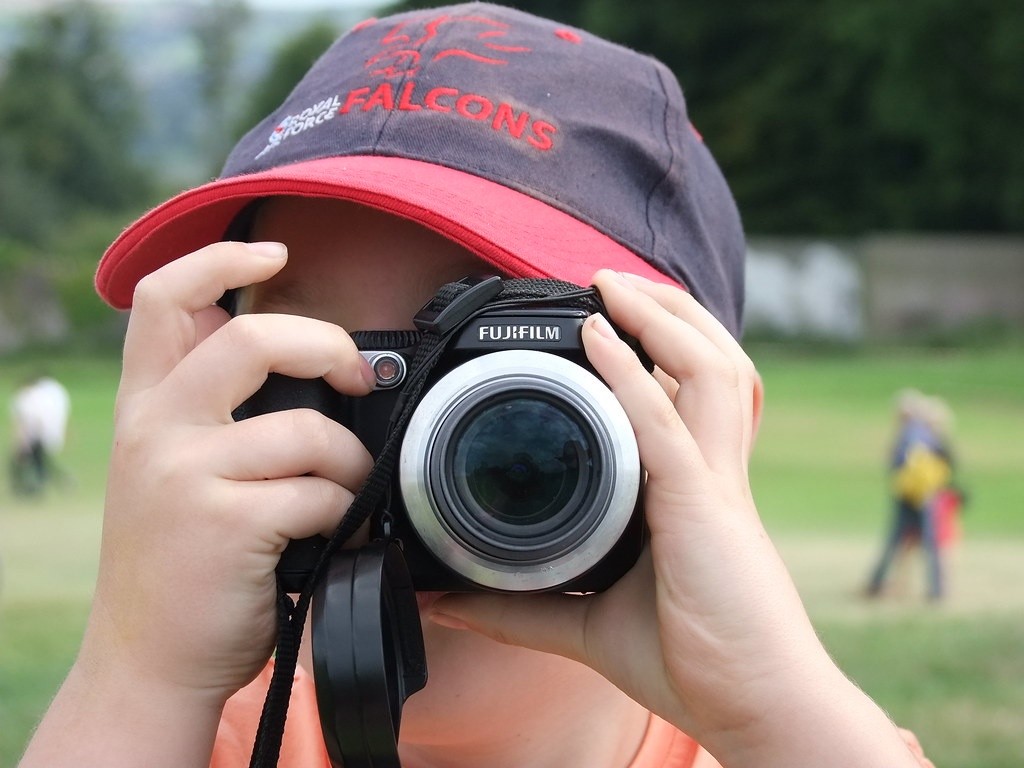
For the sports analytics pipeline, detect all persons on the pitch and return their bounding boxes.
[15,4,936,768]
[858,386,956,607]
[11,359,73,498]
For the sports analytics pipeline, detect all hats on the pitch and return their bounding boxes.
[93,1,744,407]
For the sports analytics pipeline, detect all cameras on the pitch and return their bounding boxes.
[231,300,651,595]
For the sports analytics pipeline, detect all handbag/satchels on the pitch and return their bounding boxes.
[891,443,951,512]
